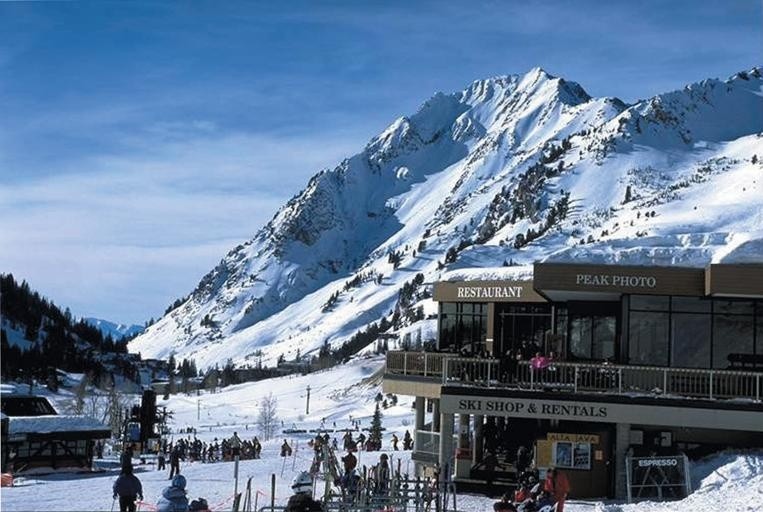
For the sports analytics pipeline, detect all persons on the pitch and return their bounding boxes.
[155,431,261,469]
[473,421,569,511]
[283,472,324,512]
[341,448,356,477]
[154,474,188,512]
[392,430,410,450]
[599,354,619,388]
[167,444,180,481]
[282,439,288,447]
[118,447,133,476]
[379,454,389,480]
[112,464,143,512]
[424,336,560,385]
[110,433,137,452]
[309,429,366,448]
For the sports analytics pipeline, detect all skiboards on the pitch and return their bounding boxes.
[309,445,344,476]
